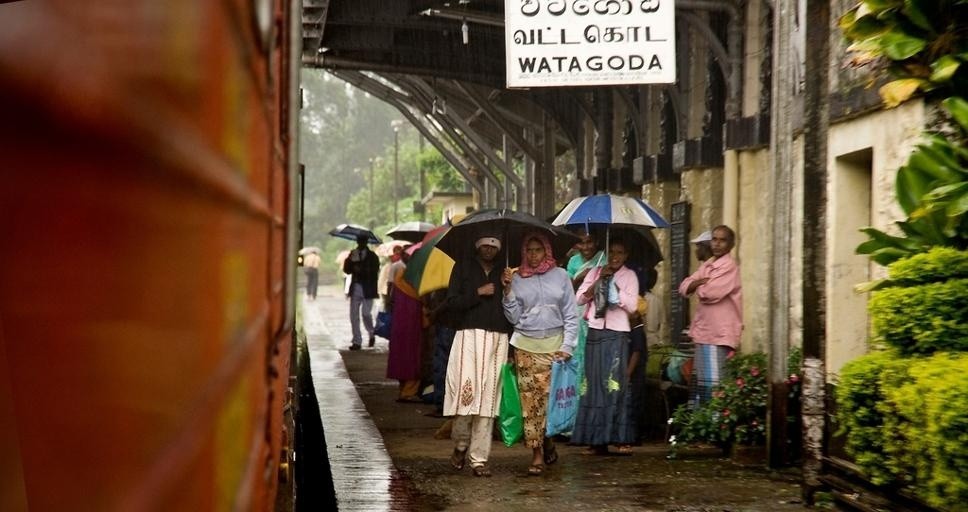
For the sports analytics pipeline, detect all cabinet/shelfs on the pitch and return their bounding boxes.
[772,383,831,471]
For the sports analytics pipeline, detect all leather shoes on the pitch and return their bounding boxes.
[349,343,360,350]
[369,337,375,347]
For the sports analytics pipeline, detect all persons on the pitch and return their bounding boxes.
[343,236,379,351]
[678,225,742,415]
[380,224,662,476]
[304,253,319,299]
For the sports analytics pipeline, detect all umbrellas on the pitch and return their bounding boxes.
[386,193,672,303]
[298,247,320,255]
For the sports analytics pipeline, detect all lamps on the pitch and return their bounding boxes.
[442,76,448,115]
[431,75,438,116]
[457,1,472,45]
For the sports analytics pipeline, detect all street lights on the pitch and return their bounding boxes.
[365,156,377,229]
[388,117,402,225]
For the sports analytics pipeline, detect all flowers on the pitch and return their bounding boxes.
[665,348,769,459]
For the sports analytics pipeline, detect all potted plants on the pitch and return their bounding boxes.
[786,346,805,383]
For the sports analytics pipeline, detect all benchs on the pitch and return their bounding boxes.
[643,375,689,443]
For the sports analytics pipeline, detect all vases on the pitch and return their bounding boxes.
[731,443,772,469]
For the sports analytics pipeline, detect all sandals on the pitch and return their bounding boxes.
[397,395,422,402]
[617,443,632,454]
[528,462,543,475]
[473,464,491,477]
[582,446,600,455]
[544,441,558,464]
[449,446,467,472]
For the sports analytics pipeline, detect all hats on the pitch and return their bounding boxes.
[475,229,504,250]
[690,231,713,242]
